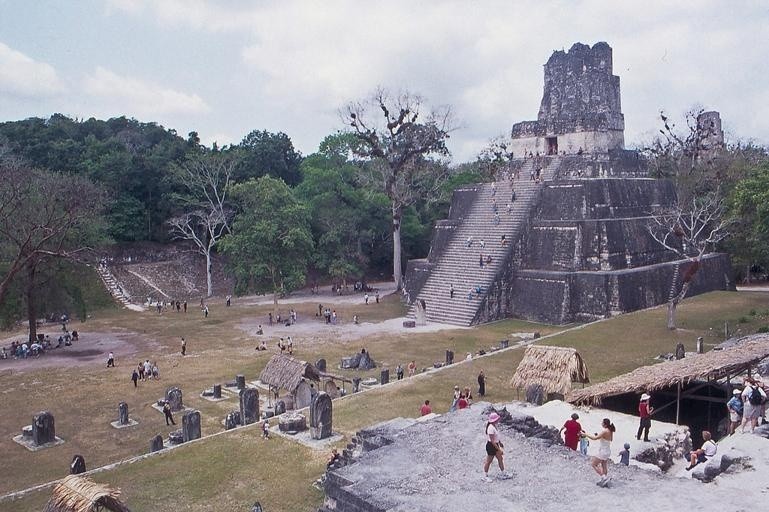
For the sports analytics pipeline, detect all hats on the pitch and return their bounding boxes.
[753,373,762,382]
[488,413,500,422]
[640,393,651,401]
[733,388,742,394]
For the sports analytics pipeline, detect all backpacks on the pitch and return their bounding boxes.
[749,389,761,405]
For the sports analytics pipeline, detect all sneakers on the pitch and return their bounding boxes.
[499,470,515,480]
[597,476,611,487]
[481,474,493,484]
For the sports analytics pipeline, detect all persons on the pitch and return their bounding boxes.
[311,279,368,296]
[144,294,209,319]
[268,308,298,327]
[559,413,615,490]
[326,447,340,471]
[0,312,81,360]
[407,359,417,377]
[419,400,431,417]
[96,251,158,271]
[270,385,281,400]
[279,337,286,354]
[258,341,268,350]
[261,415,268,437]
[477,369,485,397]
[363,292,369,305]
[263,420,271,440]
[481,412,513,484]
[634,392,654,442]
[447,143,585,302]
[225,294,232,306]
[250,502,263,512]
[395,363,404,380]
[452,385,473,410]
[130,359,160,387]
[685,430,718,472]
[617,443,631,468]
[338,387,345,397]
[256,325,263,336]
[163,400,176,426]
[725,371,768,437]
[310,384,316,404]
[286,337,294,353]
[353,313,358,325]
[315,303,338,325]
[106,351,116,368]
[178,338,188,356]
[374,291,380,303]
